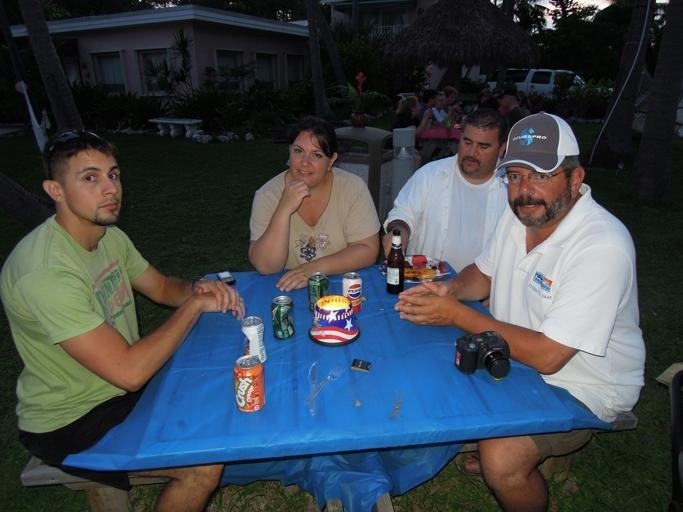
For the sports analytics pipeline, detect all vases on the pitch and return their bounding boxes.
[351,112,368,128]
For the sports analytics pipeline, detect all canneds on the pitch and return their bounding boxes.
[239,315,268,366]
[307,271,329,313]
[270,295,296,340]
[233,354,266,413]
[342,271,364,314]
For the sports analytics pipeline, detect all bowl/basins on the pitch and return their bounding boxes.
[308,294,360,347]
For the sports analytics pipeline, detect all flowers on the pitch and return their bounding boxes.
[346,71,368,111]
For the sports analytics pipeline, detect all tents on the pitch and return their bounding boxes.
[631,88,683,139]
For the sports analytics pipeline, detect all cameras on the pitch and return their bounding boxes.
[454,331,511,380]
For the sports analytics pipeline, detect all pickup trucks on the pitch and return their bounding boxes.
[486,67,614,101]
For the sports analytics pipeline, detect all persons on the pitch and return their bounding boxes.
[0,130,245,512]
[381,109,508,308]
[248,116,382,292]
[383,81,533,175]
[394,109,648,511]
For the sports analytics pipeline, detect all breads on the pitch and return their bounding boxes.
[403,265,435,280]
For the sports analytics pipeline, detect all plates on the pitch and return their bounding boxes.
[378,254,448,284]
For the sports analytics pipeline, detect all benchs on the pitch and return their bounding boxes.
[148,116,204,139]
[21,457,169,512]
[550,412,639,492]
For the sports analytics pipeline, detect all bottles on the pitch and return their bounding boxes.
[386,229,405,295]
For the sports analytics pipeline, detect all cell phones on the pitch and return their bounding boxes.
[217,271,237,284]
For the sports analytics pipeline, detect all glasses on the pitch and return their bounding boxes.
[47,130,99,180]
[500,170,565,184]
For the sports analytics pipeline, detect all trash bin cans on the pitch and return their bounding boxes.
[335,126,396,240]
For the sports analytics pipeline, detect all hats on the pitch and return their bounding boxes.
[494,111,580,174]
